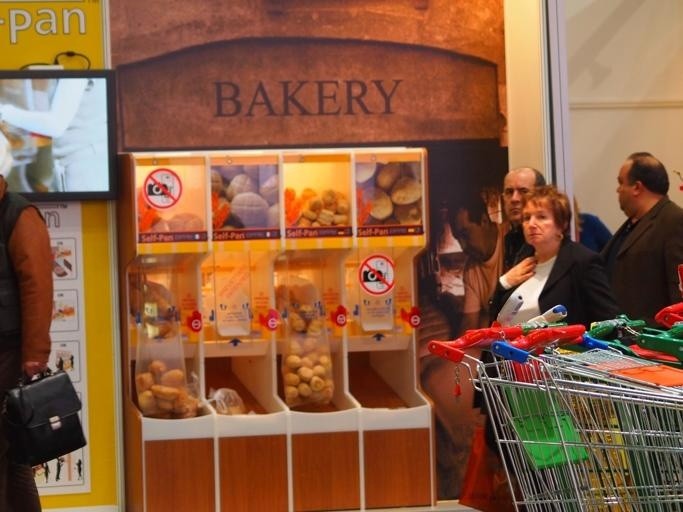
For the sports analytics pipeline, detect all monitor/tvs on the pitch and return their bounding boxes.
[0,69,121,202]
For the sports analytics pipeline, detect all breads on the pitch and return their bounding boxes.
[137,360,190,413]
[363,162,421,225]
[284,187,349,227]
[210,166,278,228]
[168,214,204,232]
[283,337,334,405]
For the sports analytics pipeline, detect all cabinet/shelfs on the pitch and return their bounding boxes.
[111,145,440,511]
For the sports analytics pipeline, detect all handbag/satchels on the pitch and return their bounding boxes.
[2,370,86,467]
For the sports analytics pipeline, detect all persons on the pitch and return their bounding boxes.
[468,185,627,512]
[571,196,614,253]
[500,165,546,276]
[416,184,507,455]
[0,173,54,511]
[594,152,682,512]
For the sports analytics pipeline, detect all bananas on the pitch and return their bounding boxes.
[135,280,181,324]
